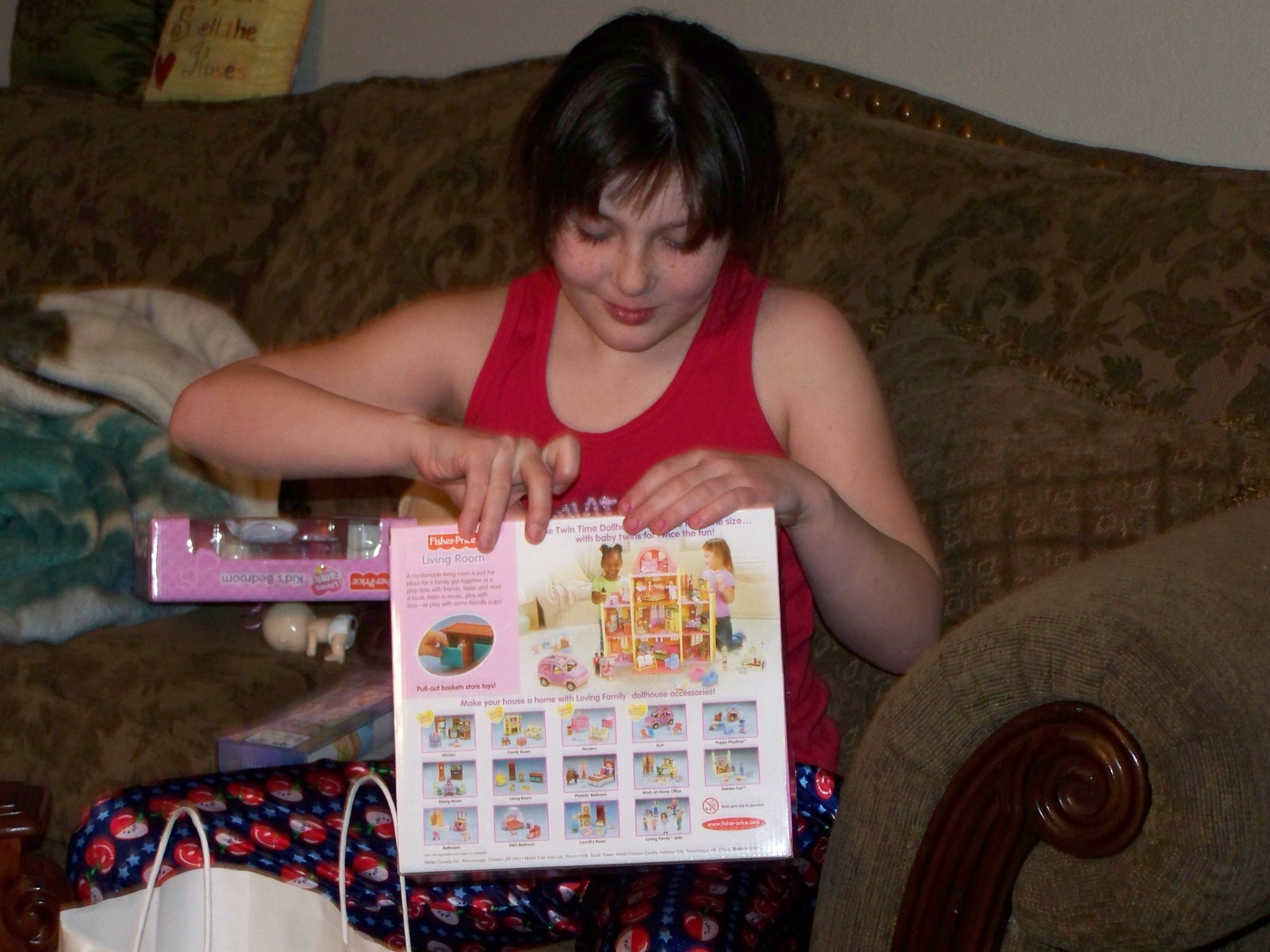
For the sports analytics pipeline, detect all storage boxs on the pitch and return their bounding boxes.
[216,671,393,773]
[133,516,420,602]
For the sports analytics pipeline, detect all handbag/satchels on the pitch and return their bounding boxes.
[58,776,411,952]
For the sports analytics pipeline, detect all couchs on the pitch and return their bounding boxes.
[0,46,1270,952]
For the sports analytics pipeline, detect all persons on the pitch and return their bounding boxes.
[590,544,631,652]
[699,537,747,653]
[74,7,942,952]
[418,629,449,657]
